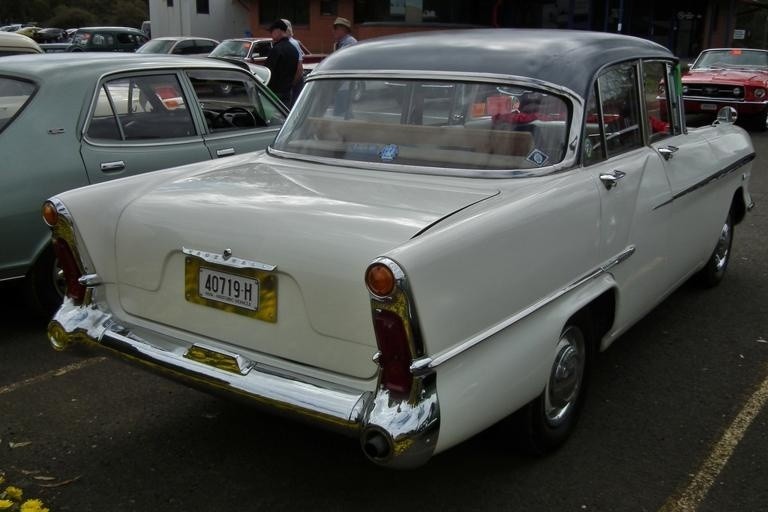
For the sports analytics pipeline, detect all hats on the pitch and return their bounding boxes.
[266,19,287,30]
[333,17,351,32]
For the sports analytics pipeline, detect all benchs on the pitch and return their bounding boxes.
[309,117,535,157]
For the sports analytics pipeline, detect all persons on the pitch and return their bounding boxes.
[332,18,358,54]
[279,17,306,105]
[265,20,303,112]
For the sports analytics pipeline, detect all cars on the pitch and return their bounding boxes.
[0,54,324,317]
[38,26,754,473]
[1,18,453,117]
[656,48,768,132]
